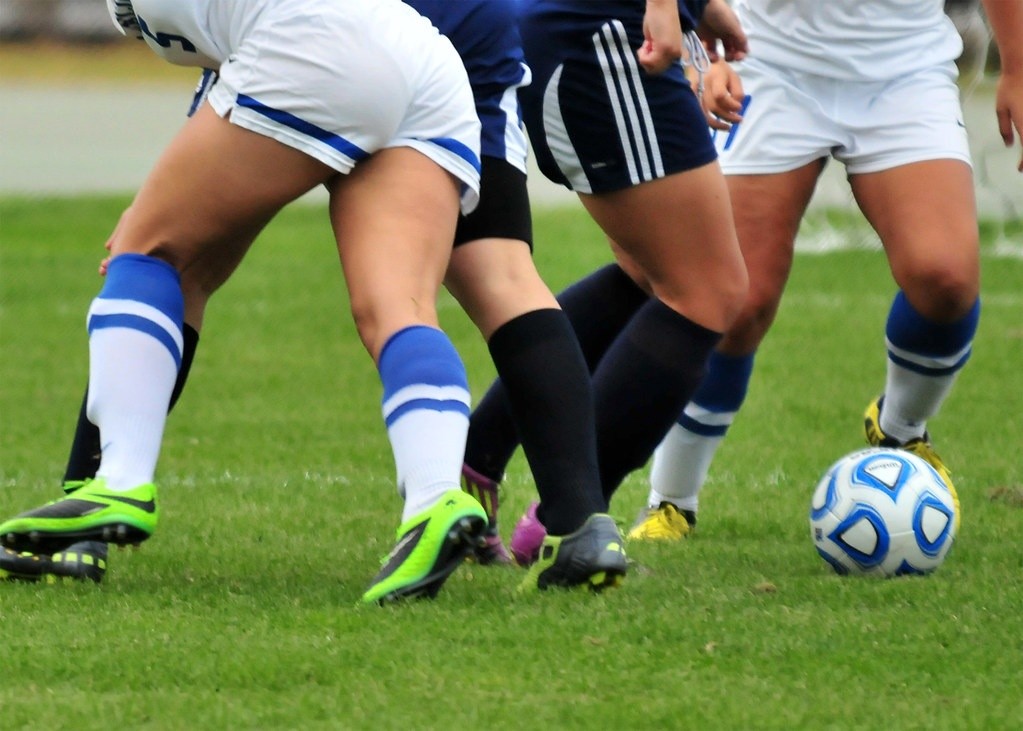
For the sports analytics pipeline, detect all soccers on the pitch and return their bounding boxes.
[809,446,958,577]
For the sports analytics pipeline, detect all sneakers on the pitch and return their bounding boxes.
[626,500,696,543]
[863,393,960,533]
[509,499,551,565]
[0,541,110,584]
[461,465,513,569]
[1,477,159,549]
[359,488,489,607]
[515,514,627,594]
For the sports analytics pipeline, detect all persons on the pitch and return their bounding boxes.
[2,1,1023,606]
